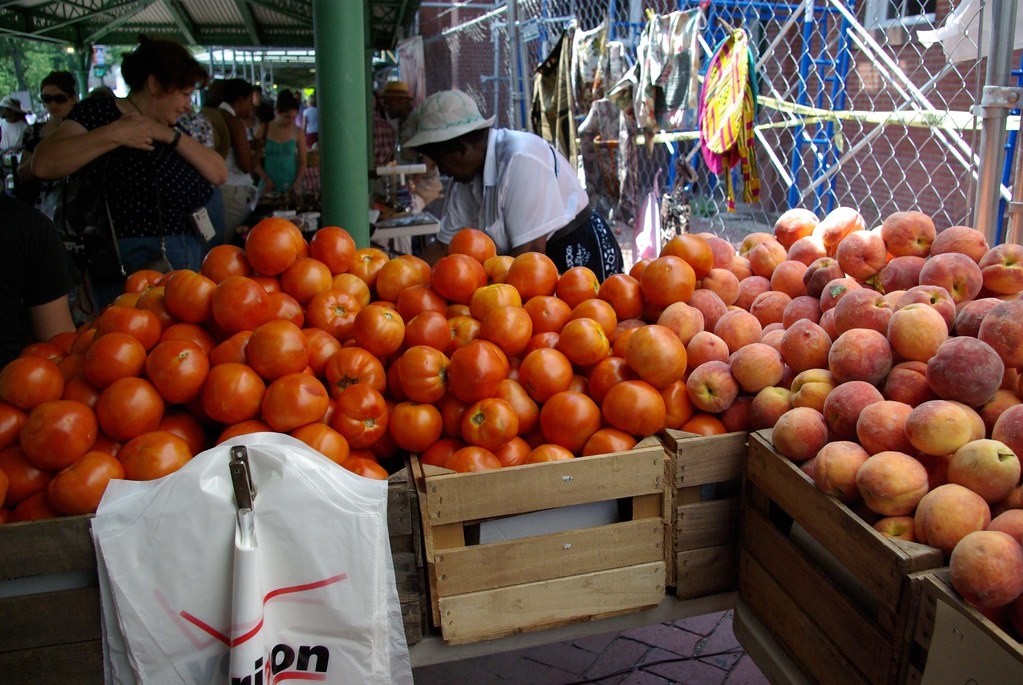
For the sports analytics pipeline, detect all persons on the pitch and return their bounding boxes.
[0,33,318,366]
[371,80,444,208]
[403,89,626,284]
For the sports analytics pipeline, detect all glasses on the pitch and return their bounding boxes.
[41,94,70,104]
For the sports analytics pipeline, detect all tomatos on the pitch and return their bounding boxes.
[0,215,726,530]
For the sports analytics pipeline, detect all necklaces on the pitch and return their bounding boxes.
[5,121,22,146]
[126,96,143,115]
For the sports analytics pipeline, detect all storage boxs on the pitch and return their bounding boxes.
[0,430,1023,685]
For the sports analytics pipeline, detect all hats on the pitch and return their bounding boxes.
[380,80,415,101]
[0,96,23,112]
[202,107,231,161]
[401,89,496,148]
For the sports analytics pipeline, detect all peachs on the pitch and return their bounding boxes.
[613,207,1023,648]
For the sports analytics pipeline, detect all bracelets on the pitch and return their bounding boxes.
[170,131,181,146]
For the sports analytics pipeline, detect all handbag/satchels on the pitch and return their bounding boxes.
[249,123,268,153]
[87,431,415,685]
[117,248,173,280]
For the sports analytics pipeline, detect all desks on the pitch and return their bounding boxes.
[371,212,441,255]
